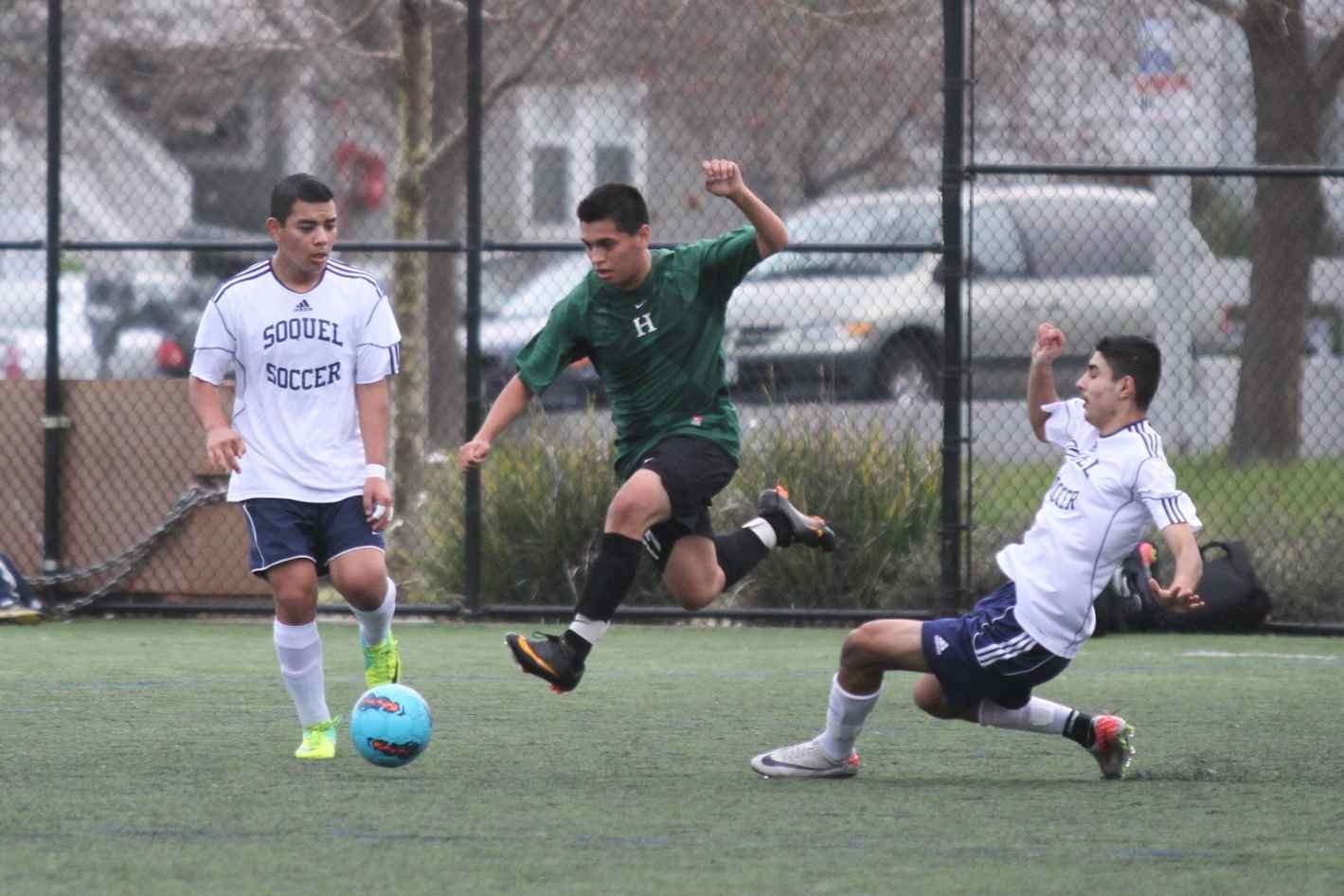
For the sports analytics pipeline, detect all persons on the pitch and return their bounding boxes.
[451,159,841,692]
[182,176,401,767]
[745,323,1209,783]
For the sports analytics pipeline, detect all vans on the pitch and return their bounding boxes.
[720,176,1221,406]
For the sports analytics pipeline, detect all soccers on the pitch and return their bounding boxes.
[350,681,431,768]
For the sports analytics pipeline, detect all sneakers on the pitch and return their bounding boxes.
[505,631,585,693]
[359,623,401,689]
[750,737,860,779]
[1086,714,1135,780]
[757,486,836,553]
[295,721,336,758]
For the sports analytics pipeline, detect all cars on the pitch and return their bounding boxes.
[1,263,172,392]
[459,237,606,416]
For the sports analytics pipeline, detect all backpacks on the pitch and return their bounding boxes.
[1194,541,1271,630]
